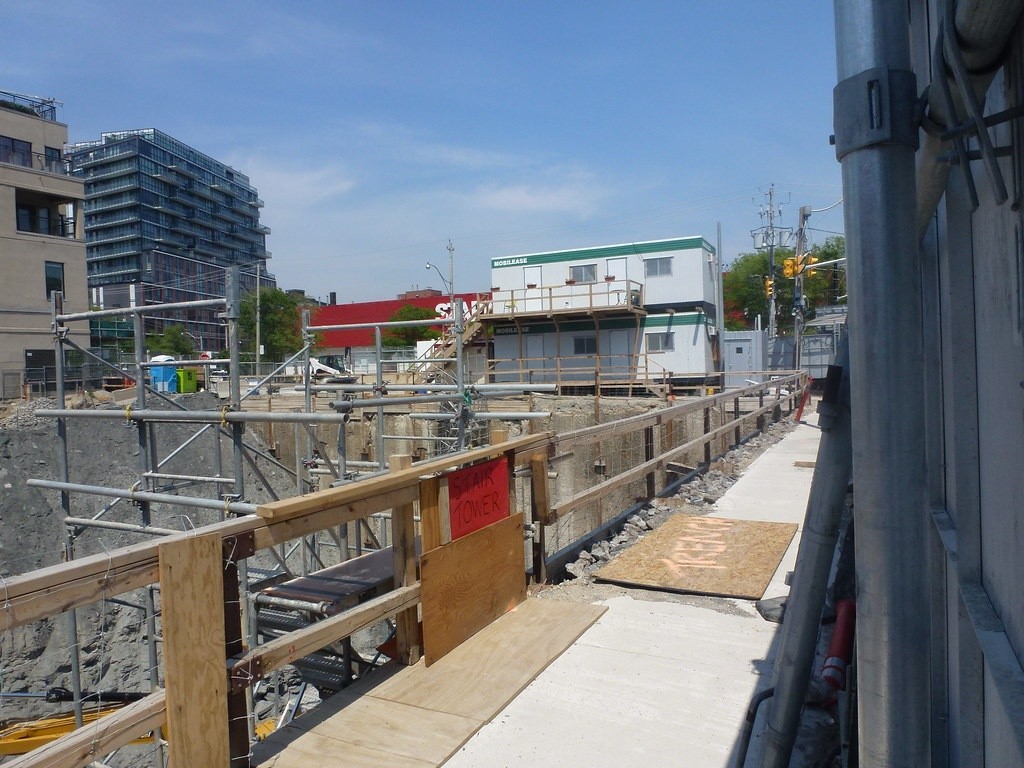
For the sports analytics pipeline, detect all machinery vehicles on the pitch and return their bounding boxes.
[306,355,358,395]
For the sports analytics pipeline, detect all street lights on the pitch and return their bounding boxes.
[426,238,456,338]
